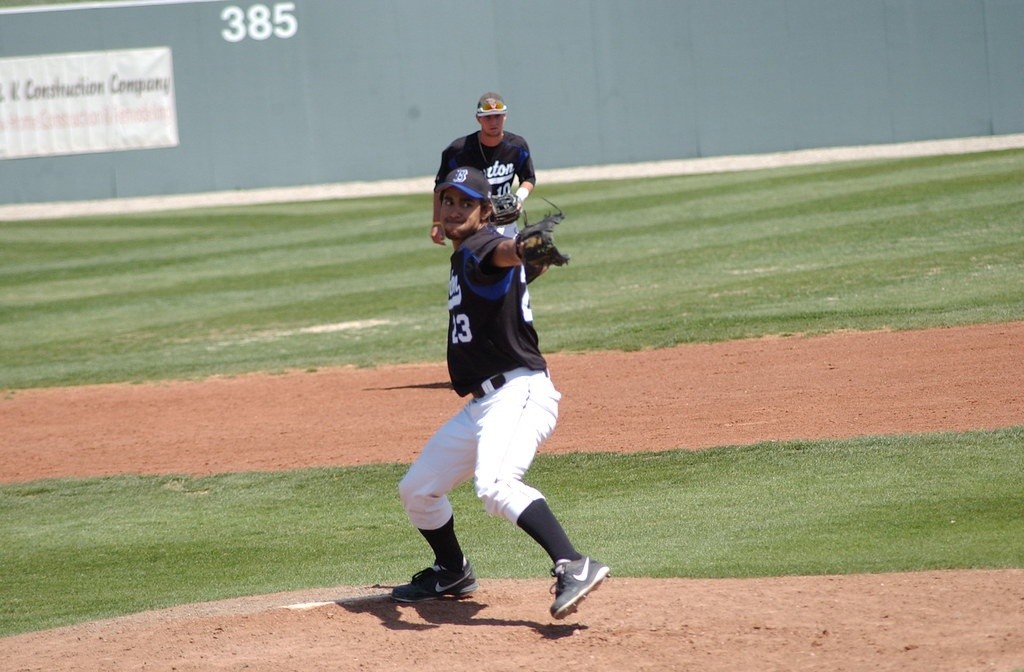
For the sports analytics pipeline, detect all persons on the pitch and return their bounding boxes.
[392,166,612,618]
[431,93,536,246]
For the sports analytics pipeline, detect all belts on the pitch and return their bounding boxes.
[470,373,506,398]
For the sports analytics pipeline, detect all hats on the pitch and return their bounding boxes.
[434,166,492,207]
[477,92,507,117]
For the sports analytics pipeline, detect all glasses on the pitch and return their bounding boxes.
[477,101,506,113]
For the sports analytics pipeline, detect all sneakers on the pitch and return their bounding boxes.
[390,559,480,602]
[549,557,610,619]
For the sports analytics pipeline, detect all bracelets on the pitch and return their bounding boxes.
[515,188,529,201]
[432,221,442,225]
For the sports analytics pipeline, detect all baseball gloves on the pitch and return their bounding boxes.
[515,211,570,265]
[491,192,521,225]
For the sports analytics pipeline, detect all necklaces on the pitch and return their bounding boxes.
[478,130,503,164]
